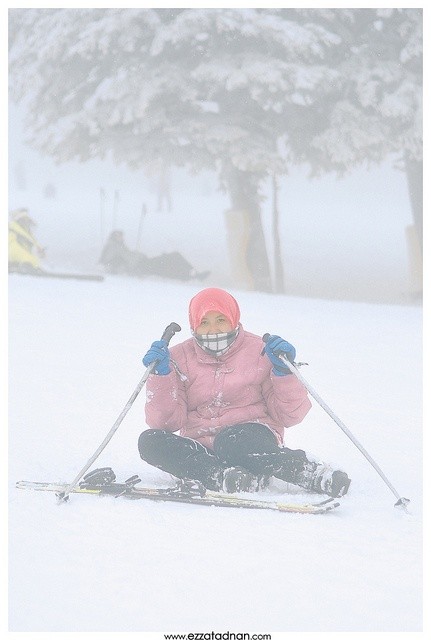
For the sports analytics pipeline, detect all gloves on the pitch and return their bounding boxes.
[142,339,173,376]
[264,335,296,377]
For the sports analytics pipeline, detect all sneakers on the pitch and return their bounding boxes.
[296,462,351,498]
[207,463,271,493]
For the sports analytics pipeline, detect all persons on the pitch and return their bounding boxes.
[98,229,194,282]
[9,209,56,278]
[77,285,351,499]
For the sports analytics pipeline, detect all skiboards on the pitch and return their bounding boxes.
[14,475,339,514]
